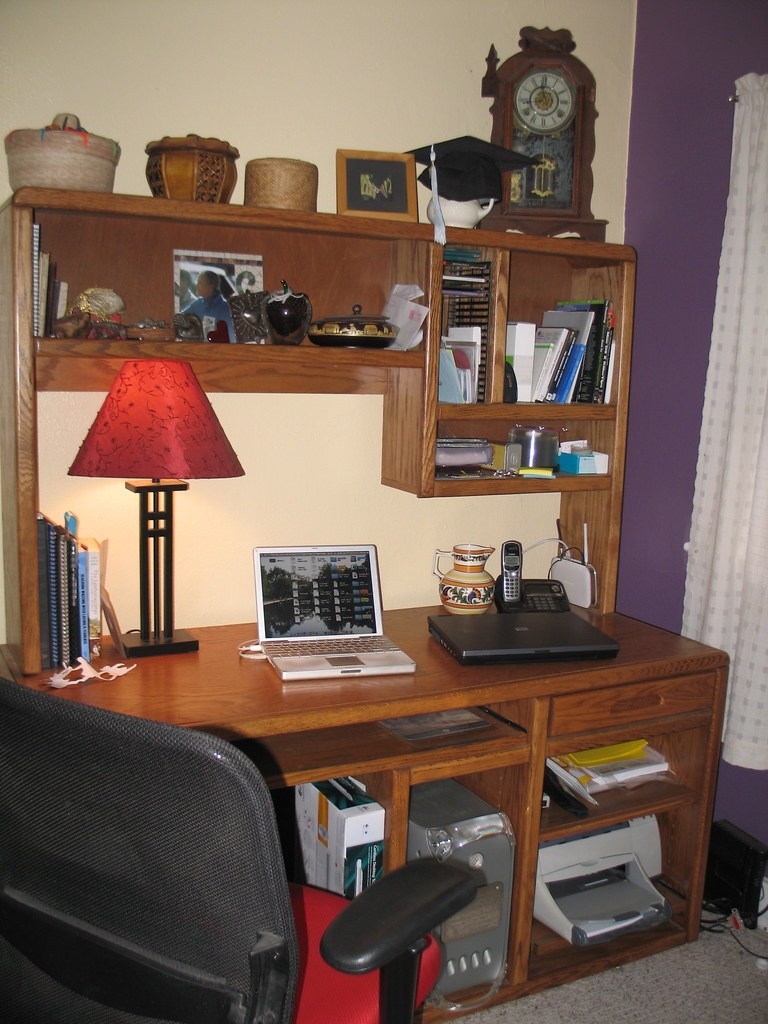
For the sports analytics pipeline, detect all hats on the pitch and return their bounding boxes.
[401,134,544,246]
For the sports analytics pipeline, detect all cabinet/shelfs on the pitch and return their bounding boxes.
[0,186,640,674]
[0,580,728,1024]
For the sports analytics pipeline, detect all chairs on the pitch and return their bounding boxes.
[0,680,485,1024]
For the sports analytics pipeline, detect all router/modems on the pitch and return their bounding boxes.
[551,519,597,608]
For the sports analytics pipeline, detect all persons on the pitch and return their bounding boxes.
[176,271,236,343]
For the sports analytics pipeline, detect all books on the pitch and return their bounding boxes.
[36,511,102,671]
[531,299,617,404]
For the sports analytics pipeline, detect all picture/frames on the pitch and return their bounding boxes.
[335,151,419,222]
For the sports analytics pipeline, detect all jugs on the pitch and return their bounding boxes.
[433,544,495,615]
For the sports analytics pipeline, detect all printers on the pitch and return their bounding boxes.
[533,813,673,947]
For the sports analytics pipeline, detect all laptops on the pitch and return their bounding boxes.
[253,544,415,681]
[427,611,619,666]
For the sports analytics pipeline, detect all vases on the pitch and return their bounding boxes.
[432,544,495,616]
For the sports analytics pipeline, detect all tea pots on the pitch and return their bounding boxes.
[426,194,495,229]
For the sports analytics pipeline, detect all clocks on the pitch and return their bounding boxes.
[480,24,608,241]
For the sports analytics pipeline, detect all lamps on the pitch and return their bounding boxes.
[68,357,244,659]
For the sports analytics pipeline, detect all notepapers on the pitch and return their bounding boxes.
[518,467,557,479]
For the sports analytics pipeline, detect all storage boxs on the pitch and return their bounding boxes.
[283,780,384,897]
[558,449,609,475]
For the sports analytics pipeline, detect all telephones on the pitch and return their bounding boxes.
[494,539,572,612]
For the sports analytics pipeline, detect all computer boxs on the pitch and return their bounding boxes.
[406,778,516,1012]
